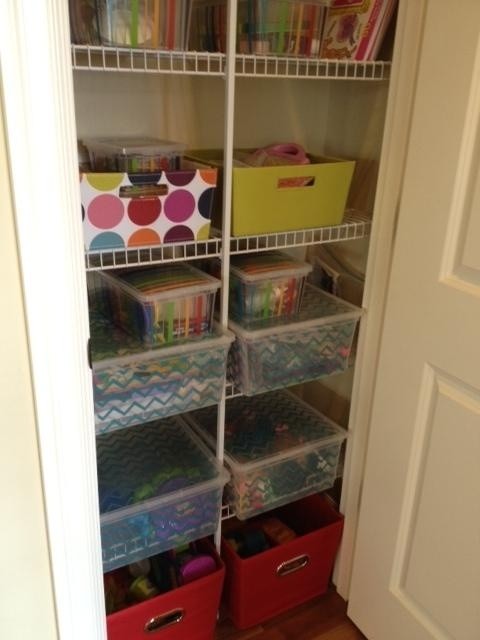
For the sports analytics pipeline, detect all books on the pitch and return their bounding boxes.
[320,0,397,65]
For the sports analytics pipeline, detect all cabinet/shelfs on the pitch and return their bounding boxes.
[66,0,397,640]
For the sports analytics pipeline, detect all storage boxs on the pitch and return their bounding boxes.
[103,538,231,640]
[188,389,347,519]
[220,283,362,398]
[236,0,396,58]
[71,0,227,52]
[92,414,229,568]
[79,135,188,171]
[80,169,220,251]
[217,248,318,324]
[191,142,360,235]
[88,319,238,433]
[88,260,220,344]
[216,491,347,625]
